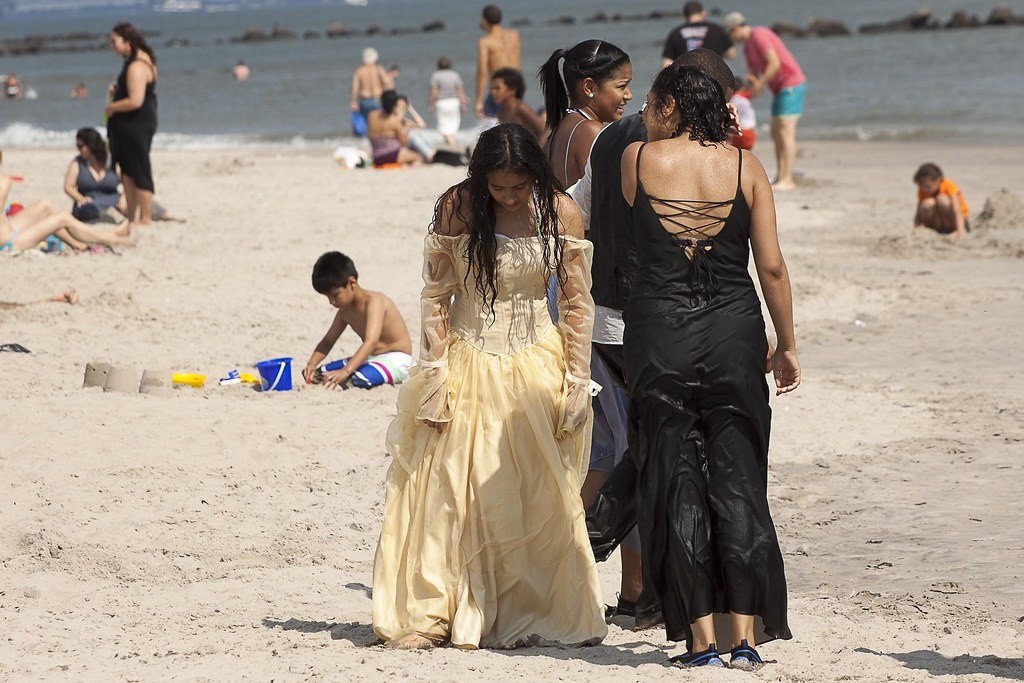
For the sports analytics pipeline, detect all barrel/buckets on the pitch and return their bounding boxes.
[255,356,293,393]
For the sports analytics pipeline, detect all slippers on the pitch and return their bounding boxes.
[0,343,31,354]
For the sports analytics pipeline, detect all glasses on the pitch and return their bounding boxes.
[77,143,86,149]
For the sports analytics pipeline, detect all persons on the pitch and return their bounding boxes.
[0,153,141,252]
[232,63,251,82]
[912,163,971,238]
[659,1,809,192]
[349,4,548,169]
[535,39,801,671]
[74,82,86,97]
[302,251,413,390]
[370,124,604,651]
[4,73,22,100]
[106,23,157,226]
[63,128,186,226]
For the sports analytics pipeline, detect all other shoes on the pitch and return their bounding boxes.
[76,246,105,255]
[668,642,725,669]
[730,638,762,669]
[604,592,635,618]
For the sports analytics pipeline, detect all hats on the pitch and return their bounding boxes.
[721,11,745,34]
[362,47,378,64]
